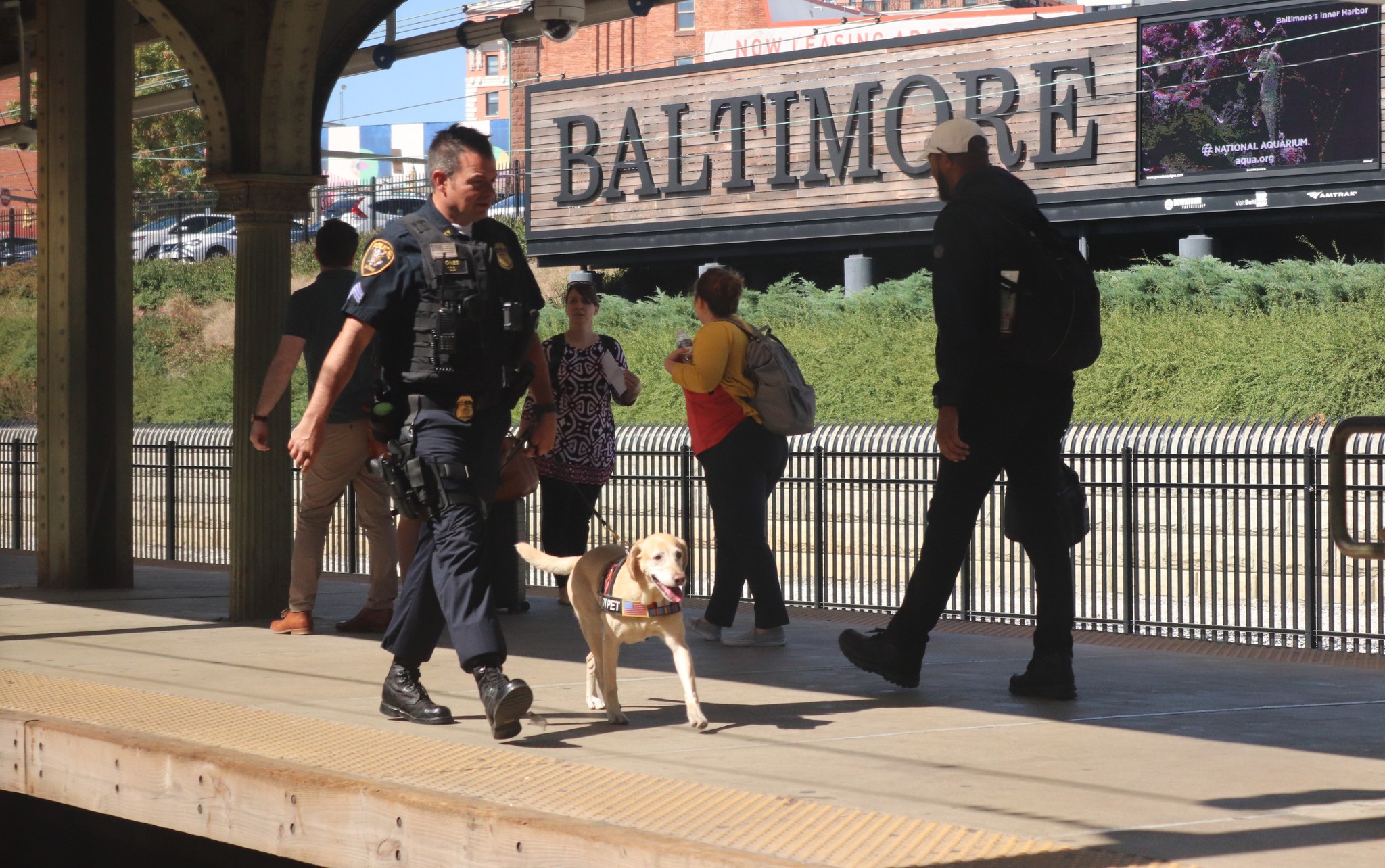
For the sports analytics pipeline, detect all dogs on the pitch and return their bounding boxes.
[510,528,709,733]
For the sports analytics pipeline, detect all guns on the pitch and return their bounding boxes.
[364,457,419,520]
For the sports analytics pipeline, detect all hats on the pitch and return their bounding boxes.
[915,118,989,163]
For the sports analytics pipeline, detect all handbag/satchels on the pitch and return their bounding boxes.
[1003,463,1089,548]
[496,431,539,502]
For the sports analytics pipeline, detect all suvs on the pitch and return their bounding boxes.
[321,192,427,240]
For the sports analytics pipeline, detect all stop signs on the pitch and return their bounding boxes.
[0,187,12,207]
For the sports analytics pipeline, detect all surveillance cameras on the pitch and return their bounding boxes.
[534,0,586,43]
[18,144,30,151]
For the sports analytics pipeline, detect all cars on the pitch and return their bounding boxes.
[132,213,310,265]
[0,238,37,266]
[291,222,322,245]
[488,194,525,226]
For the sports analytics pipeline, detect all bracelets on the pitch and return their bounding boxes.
[531,399,558,418]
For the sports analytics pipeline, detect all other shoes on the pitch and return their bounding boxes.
[687,617,722,641]
[558,597,570,605]
[723,626,786,646]
[838,619,925,688]
[1009,642,1078,701]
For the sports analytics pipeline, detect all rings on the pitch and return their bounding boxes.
[630,384,633,387]
[252,441,254,443]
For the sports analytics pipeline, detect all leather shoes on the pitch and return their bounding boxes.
[269,607,314,635]
[335,608,394,633]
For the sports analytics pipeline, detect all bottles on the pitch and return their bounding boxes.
[675,328,693,365]
[999,272,1019,334]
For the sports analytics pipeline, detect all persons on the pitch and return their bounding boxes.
[249,219,420,633]
[517,282,642,604]
[663,268,788,646]
[839,117,1077,704]
[288,122,558,739]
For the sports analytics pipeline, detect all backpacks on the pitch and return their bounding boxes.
[997,227,1102,371]
[713,318,815,436]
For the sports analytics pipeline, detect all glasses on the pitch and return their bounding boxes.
[924,139,952,159]
[567,281,597,295]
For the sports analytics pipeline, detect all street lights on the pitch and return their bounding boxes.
[340,84,347,124]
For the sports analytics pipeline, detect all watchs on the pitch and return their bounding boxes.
[250,413,268,423]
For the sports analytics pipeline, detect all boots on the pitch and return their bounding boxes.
[379,654,455,724]
[470,653,533,741]
